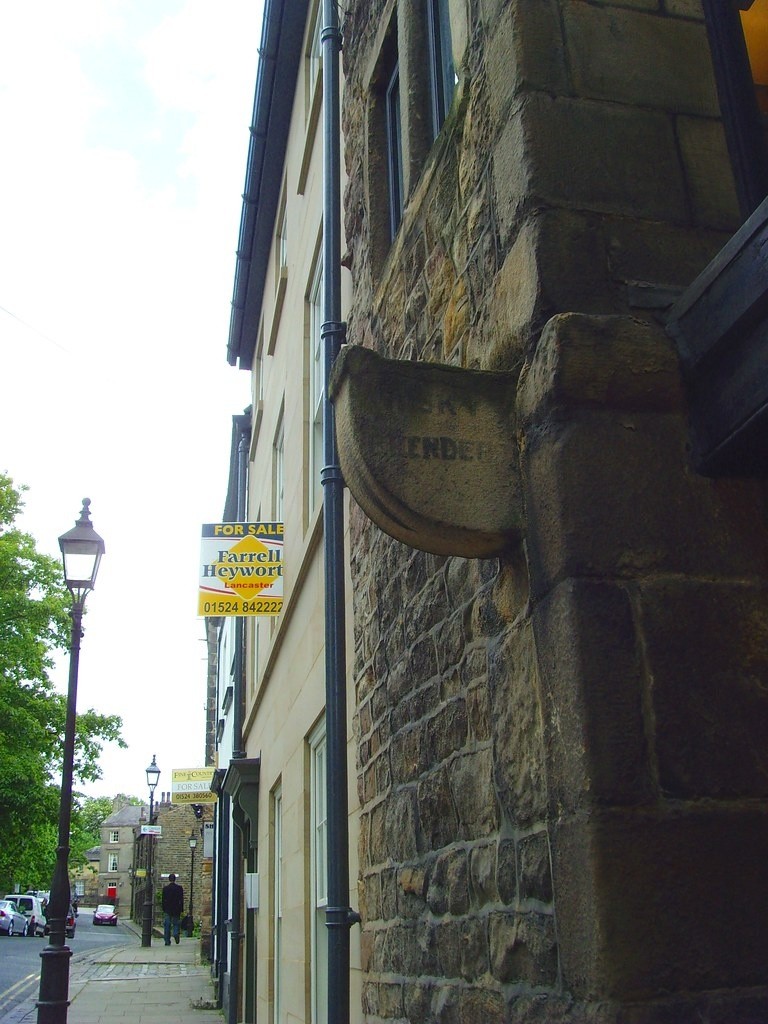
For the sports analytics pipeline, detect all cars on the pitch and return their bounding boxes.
[93,904,119,926]
[26,891,78,938]
[0,900,28,937]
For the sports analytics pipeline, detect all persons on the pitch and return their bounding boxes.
[72,901,77,912]
[162,874,184,945]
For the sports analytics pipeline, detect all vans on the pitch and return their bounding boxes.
[4,895,47,937]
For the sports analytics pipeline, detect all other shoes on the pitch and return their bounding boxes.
[165,942,170,946]
[175,935,179,944]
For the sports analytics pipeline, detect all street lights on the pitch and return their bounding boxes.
[186,830,196,938]
[141,754,162,948]
[35,498,106,1024]
